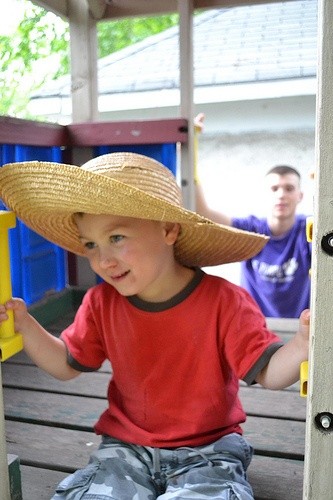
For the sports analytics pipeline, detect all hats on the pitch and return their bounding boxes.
[0,151,271,267]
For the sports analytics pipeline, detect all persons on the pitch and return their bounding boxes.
[194,113,313,318]
[0,152,310,500]
